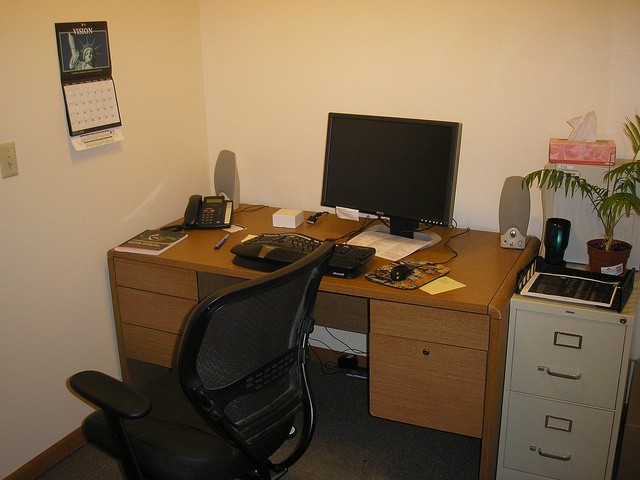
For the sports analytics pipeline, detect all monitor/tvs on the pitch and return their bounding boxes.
[320,113,462,251]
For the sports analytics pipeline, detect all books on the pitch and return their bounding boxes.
[115,227,189,255]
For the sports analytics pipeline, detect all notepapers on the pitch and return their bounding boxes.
[270,206,307,230]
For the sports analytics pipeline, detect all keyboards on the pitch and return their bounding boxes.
[230,232,376,270]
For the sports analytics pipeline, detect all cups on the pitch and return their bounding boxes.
[542,218,572,266]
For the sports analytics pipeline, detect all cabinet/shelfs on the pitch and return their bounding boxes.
[497,265,640,478]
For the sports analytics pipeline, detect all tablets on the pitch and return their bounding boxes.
[520,272,617,307]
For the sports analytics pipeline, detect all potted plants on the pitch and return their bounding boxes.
[520,117,640,273]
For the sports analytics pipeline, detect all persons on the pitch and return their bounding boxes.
[67,33,102,71]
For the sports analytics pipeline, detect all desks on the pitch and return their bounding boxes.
[105,202,541,479]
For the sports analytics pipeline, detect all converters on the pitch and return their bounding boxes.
[337,356,358,369]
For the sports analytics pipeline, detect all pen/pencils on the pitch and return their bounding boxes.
[213,233,230,249]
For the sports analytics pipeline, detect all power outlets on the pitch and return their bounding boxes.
[0,142,18,179]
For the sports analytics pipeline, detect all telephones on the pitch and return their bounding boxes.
[160,195,233,232]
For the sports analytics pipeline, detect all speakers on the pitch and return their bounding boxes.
[498,176,530,249]
[214,149,240,209]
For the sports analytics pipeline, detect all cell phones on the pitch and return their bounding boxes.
[345,367,368,380]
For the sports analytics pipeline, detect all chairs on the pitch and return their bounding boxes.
[64,242,337,480]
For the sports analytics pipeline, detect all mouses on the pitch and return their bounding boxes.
[391,264,414,280]
